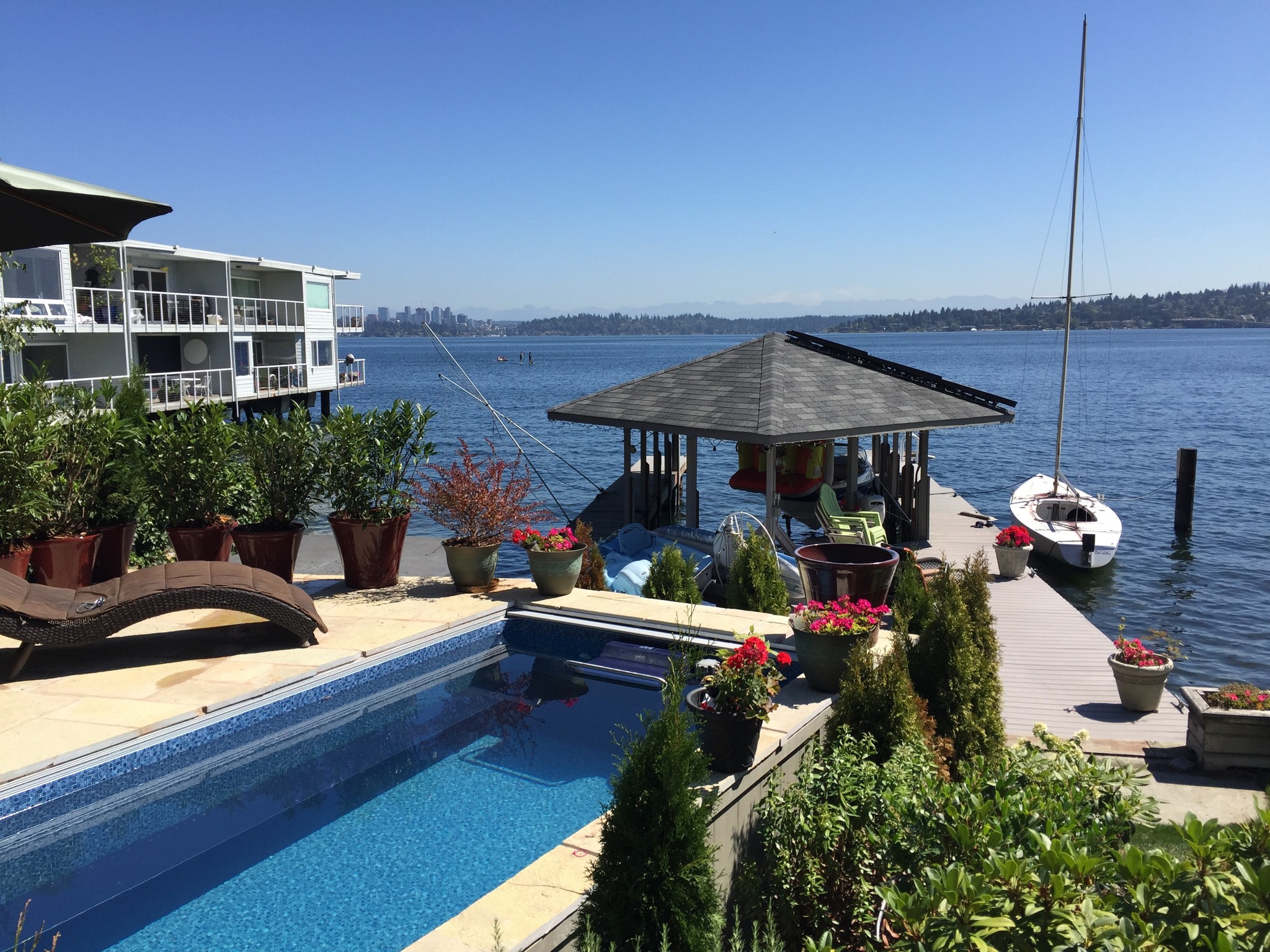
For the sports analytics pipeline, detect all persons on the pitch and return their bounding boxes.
[520,351,523,363]
[502,356,505,360]
[498,356,500,360]
[529,352,532,363]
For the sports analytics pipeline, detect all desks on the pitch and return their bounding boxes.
[172,299,202,322]
[170,377,201,398]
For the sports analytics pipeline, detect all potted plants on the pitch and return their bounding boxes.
[351,317,360,327]
[0,365,559,594]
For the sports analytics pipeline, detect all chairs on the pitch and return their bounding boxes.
[603,520,728,599]
[0,560,323,680]
[193,376,214,399]
[270,363,293,391]
[815,484,951,594]
[156,377,164,400]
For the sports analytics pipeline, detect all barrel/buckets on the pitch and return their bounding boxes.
[794,542,900,649]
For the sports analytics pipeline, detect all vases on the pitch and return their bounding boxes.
[1108,653,1173,710]
[527,542,588,594]
[1180,687,1270,772]
[789,612,879,695]
[993,543,1033,577]
[795,543,898,624]
[687,687,765,772]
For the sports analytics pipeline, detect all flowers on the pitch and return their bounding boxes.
[1208,682,1270,711]
[793,595,891,633]
[996,526,1035,548]
[512,526,578,551]
[1113,616,1187,666]
[697,631,791,722]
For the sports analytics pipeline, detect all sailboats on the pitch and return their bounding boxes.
[1011,13,1124,569]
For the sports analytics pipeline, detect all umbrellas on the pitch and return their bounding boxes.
[0,159,173,253]
